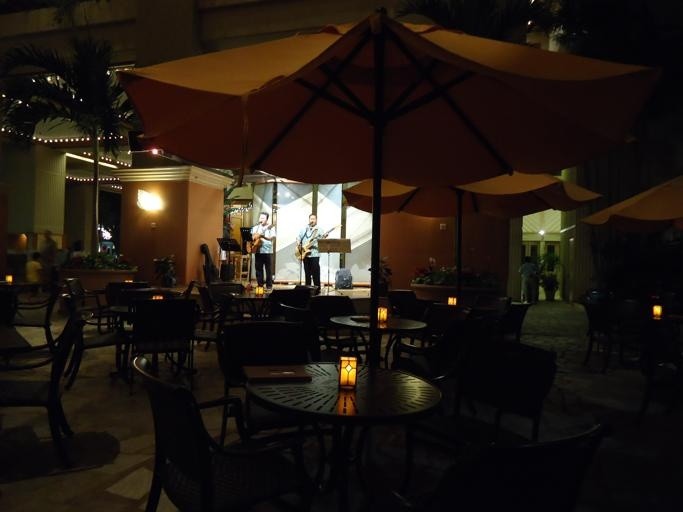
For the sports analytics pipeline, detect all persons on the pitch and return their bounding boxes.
[249,212,275,291]
[25,252,45,290]
[296,214,329,294]
[38,229,58,265]
[517,255,536,304]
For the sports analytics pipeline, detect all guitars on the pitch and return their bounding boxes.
[246,222,278,253]
[295,223,343,261]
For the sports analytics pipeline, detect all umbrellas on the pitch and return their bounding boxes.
[343,172,602,304]
[112,6,654,365]
[582,174,683,237]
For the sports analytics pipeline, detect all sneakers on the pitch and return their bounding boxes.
[255,285,272,289]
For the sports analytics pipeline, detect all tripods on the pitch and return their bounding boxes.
[320,252,344,296]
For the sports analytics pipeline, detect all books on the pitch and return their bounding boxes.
[243,363,311,384]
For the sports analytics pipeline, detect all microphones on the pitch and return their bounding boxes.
[309,221,313,226]
[259,221,262,224]
